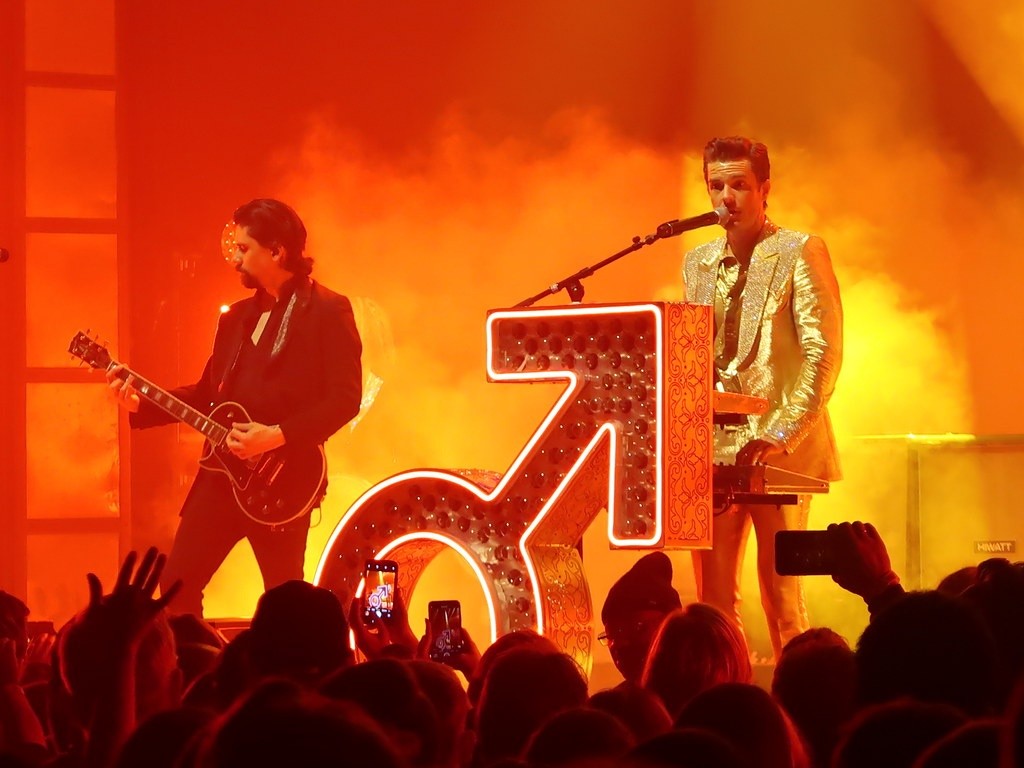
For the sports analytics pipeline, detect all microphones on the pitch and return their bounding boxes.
[657,208,728,238]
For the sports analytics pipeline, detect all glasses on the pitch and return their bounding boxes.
[597,610,668,645]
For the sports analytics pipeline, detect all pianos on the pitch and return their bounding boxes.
[713,461,829,512]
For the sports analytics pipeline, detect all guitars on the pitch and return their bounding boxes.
[67,326,329,526]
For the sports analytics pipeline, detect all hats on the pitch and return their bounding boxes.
[251,578,346,683]
[601,550,680,624]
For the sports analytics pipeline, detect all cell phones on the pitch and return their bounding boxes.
[362,560,398,625]
[775,531,846,575]
[428,600,463,668]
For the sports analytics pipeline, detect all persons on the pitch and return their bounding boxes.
[0,521,1024,768]
[684,135,843,665]
[107,199,362,619]
